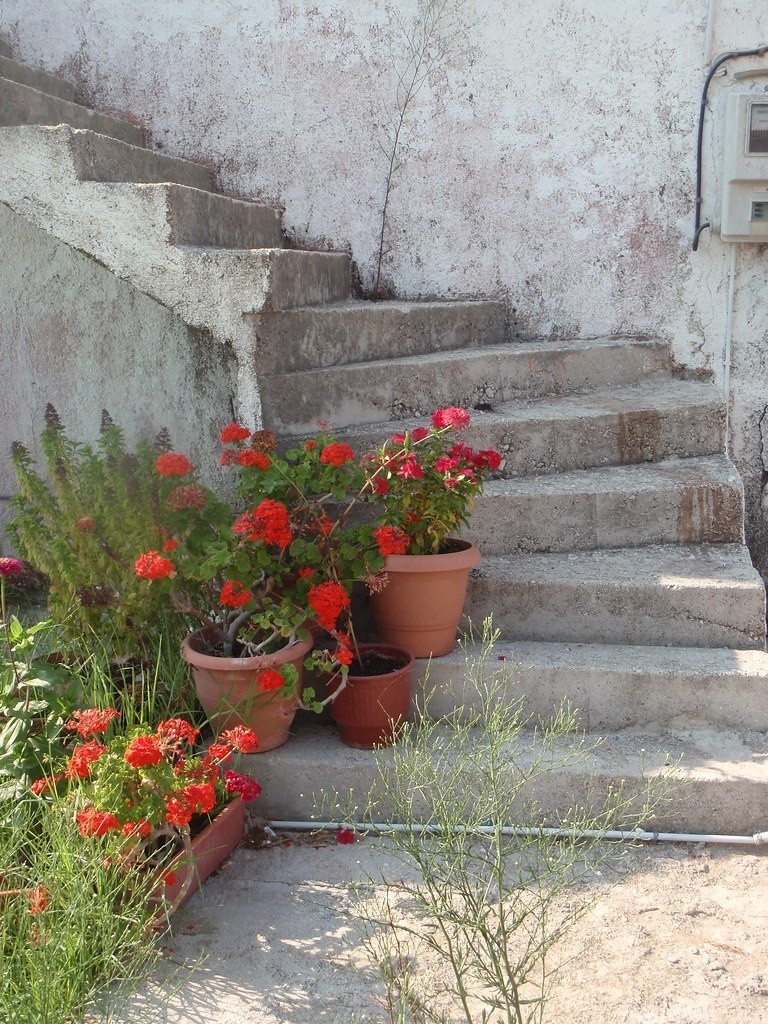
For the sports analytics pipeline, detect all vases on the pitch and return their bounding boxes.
[270,564,320,640]
[181,620,313,753]
[107,796,245,919]
[318,644,416,751]
[357,535,481,659]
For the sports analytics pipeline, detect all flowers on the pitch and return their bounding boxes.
[29,406,501,949]
